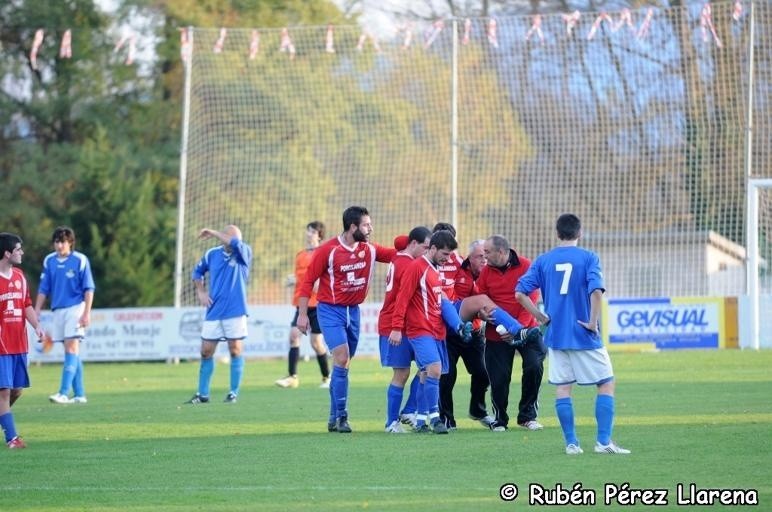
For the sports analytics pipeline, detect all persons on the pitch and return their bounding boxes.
[186,223,253,404]
[378,223,544,434]
[0,233,45,448]
[35,226,95,404]
[514,213,633,454]
[275,221,331,388]
[296,207,397,433]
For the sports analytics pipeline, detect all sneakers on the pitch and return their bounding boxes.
[415,424,434,434]
[69,396,88,405]
[400,412,417,426]
[467,411,495,427]
[516,420,544,431]
[336,417,353,433]
[353,420,411,432]
[458,320,474,344]
[593,439,633,456]
[327,422,338,432]
[446,424,458,431]
[6,437,28,450]
[509,325,541,348]
[564,442,584,455]
[492,424,506,432]
[48,393,71,404]
[430,421,448,434]
[319,377,331,389]
[275,375,300,388]
[183,392,210,406]
[223,392,240,404]
